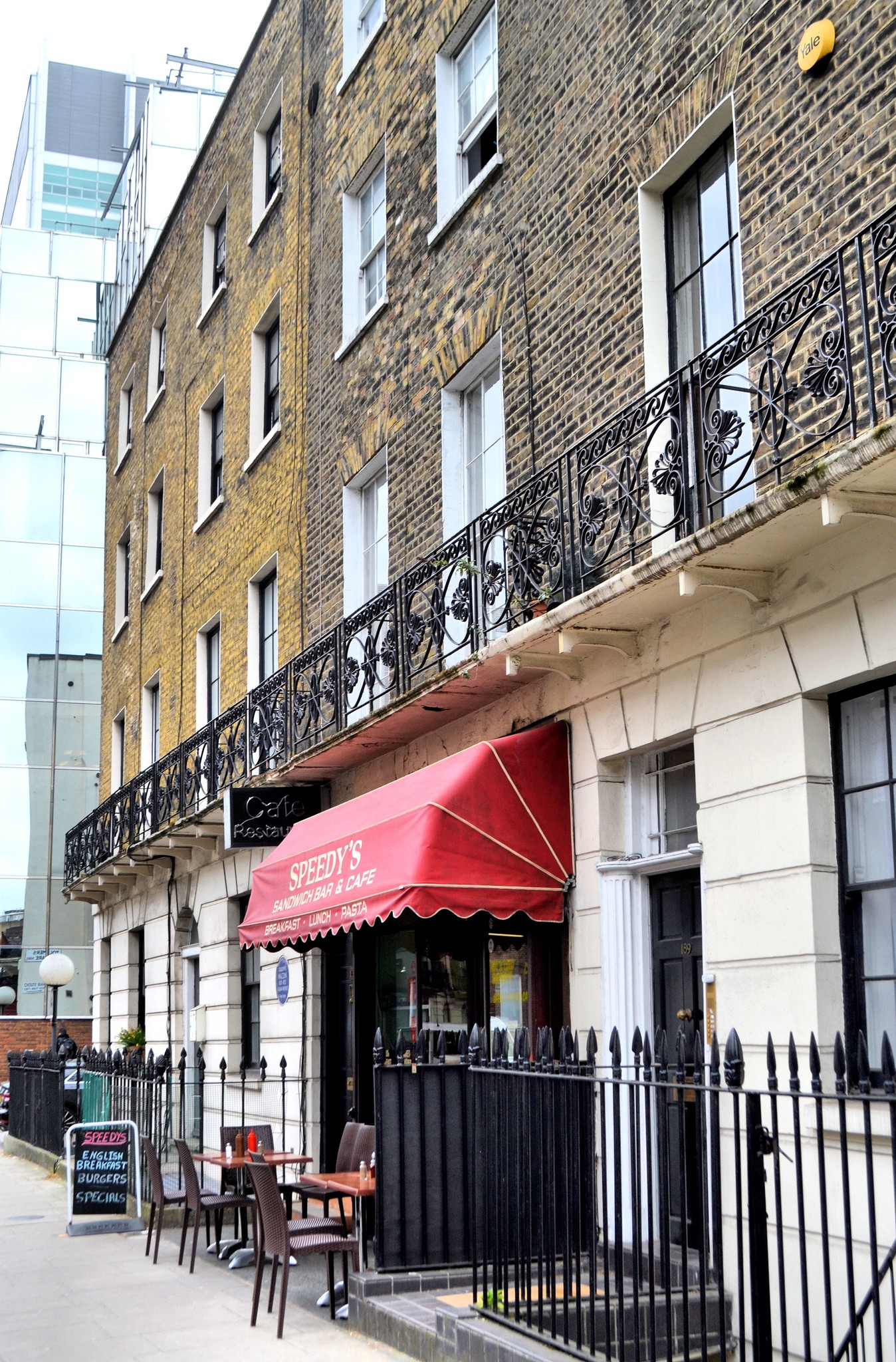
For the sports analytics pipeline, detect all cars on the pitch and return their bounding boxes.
[1,1063,92,1143]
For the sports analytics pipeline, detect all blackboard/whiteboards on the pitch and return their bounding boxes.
[73,1129,129,1215]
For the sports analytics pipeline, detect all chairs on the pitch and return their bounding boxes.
[286,1121,365,1219]
[217,1125,295,1240]
[301,1125,376,1227]
[243,1158,361,1341]
[173,1137,259,1274]
[139,1133,217,1264]
[246,1149,352,1319]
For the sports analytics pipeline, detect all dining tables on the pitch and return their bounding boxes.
[299,1171,376,1308]
[328,1176,375,1320]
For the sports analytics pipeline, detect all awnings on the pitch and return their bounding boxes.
[236,719,577,955]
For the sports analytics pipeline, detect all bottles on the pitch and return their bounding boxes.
[370,1151,376,1178]
[248,1129,256,1152]
[257,1140,264,1157]
[360,1160,368,1180]
[236,1128,245,1157]
[226,1142,233,1158]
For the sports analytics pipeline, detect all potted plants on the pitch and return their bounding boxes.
[116,1025,145,1062]
[415,553,562,621]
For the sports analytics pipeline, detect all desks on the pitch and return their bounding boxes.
[191,1148,293,1260]
[210,1153,314,1269]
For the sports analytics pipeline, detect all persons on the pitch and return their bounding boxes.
[46,1028,81,1061]
